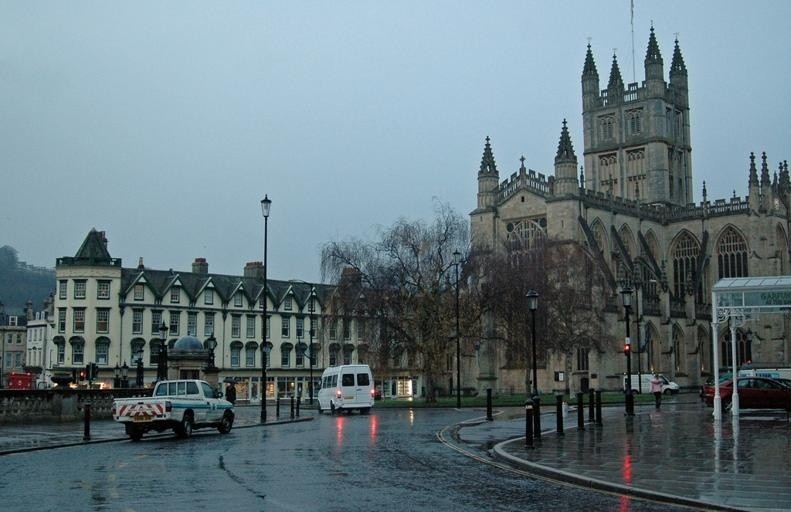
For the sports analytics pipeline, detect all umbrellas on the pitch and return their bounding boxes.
[224,378,238,384]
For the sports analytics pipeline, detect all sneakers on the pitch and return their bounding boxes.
[656,408,662,412]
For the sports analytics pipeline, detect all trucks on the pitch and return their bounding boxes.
[9,373,37,389]
[623,372,682,397]
[319,364,375,416]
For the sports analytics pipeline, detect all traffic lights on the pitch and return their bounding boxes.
[79,369,85,382]
[93,363,99,379]
[86,363,91,381]
[624,344,630,356]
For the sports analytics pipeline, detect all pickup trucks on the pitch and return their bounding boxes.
[112,380,236,439]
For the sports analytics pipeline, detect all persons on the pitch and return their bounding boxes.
[651,375,662,409]
[225,383,235,403]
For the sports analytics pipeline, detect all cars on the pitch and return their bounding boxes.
[374,391,382,400]
[699,368,791,413]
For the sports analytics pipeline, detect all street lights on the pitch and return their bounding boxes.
[206,331,220,371]
[260,195,273,423]
[157,319,170,382]
[527,286,542,439]
[121,360,129,388]
[453,249,462,409]
[114,359,121,388]
[619,272,634,416]
[134,345,146,388]
[288,277,317,405]
